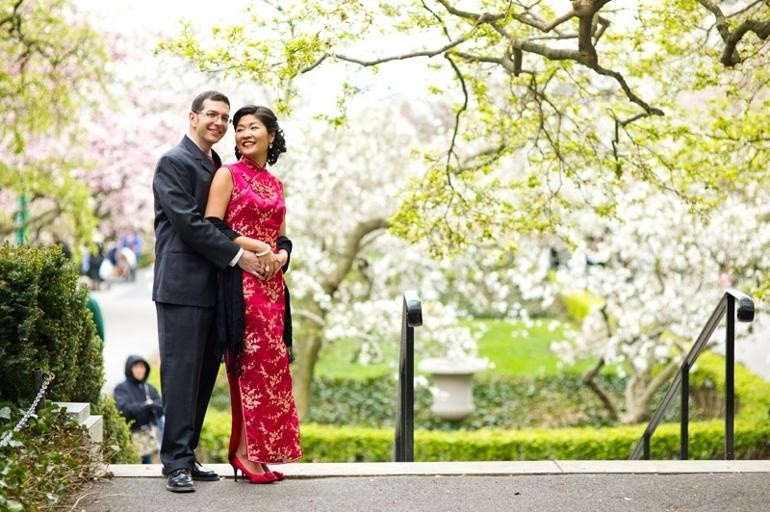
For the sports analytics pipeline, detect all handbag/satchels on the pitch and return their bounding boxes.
[131,424,160,456]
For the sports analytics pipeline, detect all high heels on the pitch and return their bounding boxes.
[230,453,278,484]
[241,462,284,481]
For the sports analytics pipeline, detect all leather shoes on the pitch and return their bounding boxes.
[167,468,196,492]
[192,464,219,481]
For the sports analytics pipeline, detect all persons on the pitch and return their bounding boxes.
[51,220,141,290]
[203,105,304,486]
[114,355,165,464]
[152,90,267,494]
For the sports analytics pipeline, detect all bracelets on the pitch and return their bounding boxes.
[255,244,272,257]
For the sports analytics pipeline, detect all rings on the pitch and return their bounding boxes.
[265,266,270,271]
